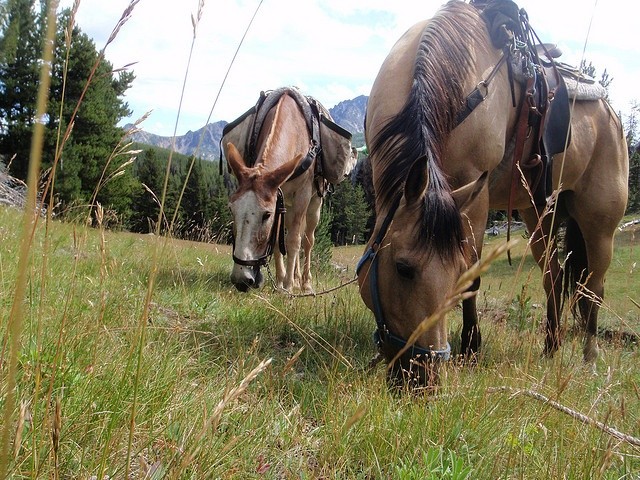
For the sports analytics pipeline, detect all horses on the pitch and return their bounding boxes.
[357,0,630,411]
[219,92,331,295]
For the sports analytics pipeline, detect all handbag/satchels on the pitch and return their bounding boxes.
[320,115,357,185]
[222,106,257,175]
